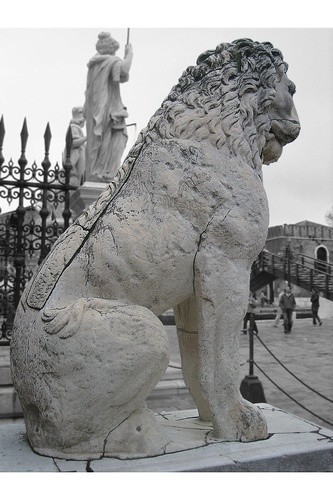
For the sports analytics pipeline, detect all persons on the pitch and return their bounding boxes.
[82,31,134,184]
[309,287,322,326]
[62,106,87,182]
[242,285,297,335]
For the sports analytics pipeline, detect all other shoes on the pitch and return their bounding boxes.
[241,330,247,334]
[256,333,257,335]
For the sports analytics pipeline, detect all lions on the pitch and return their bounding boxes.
[10,38,301,461]
